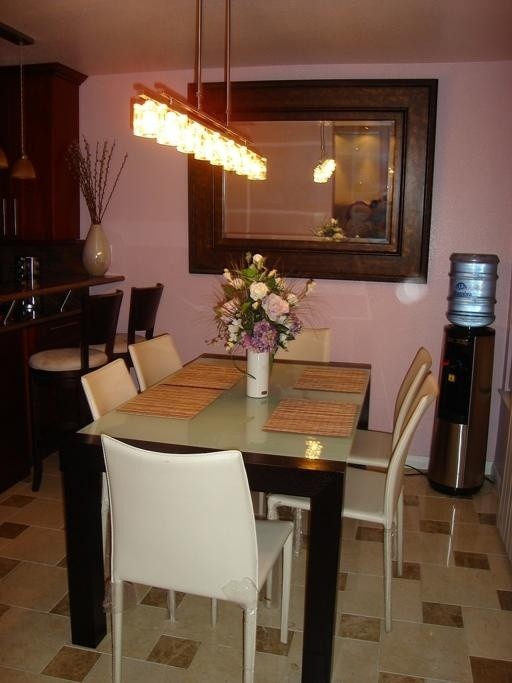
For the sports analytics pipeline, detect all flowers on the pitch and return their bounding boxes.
[198,253,318,378]
[306,218,345,238]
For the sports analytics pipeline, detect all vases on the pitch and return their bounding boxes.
[245,350,271,399]
[81,222,112,276]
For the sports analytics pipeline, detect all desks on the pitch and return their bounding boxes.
[0,274,124,496]
[77,352,371,682]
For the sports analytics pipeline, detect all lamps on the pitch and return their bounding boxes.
[130,0,268,182]
[312,121,336,184]
[0,23,37,201]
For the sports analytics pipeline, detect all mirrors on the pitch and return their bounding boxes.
[187,78,439,284]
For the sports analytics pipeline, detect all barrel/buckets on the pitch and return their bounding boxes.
[446,253,500,327]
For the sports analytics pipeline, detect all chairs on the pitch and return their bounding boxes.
[272,328,333,365]
[100,432,293,682]
[294,347,433,576]
[81,358,137,561]
[267,374,437,632]
[87,282,163,367]
[128,334,185,390]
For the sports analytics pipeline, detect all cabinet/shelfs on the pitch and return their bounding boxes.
[0,62,88,278]
